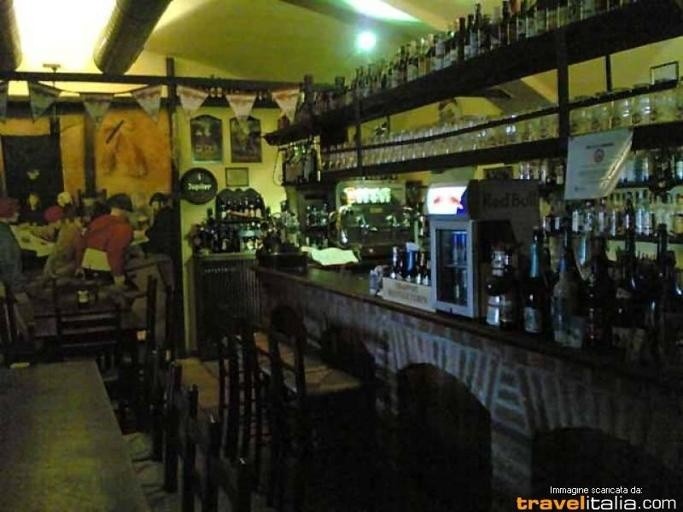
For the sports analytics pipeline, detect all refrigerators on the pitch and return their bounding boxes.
[426,179,542,320]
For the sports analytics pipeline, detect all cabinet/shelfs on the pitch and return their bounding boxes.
[264,0,683,183]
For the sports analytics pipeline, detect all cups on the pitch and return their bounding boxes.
[317,75,683,171]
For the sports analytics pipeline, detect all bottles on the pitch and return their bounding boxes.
[290,0,635,124]
[206,196,266,253]
[483,223,683,370]
[516,142,683,237]
[388,241,430,287]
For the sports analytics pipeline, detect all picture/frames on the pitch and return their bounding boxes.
[649,61,679,83]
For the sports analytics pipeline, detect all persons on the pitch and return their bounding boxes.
[0,186,179,368]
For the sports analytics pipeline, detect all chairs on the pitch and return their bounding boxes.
[0,258,367,512]
